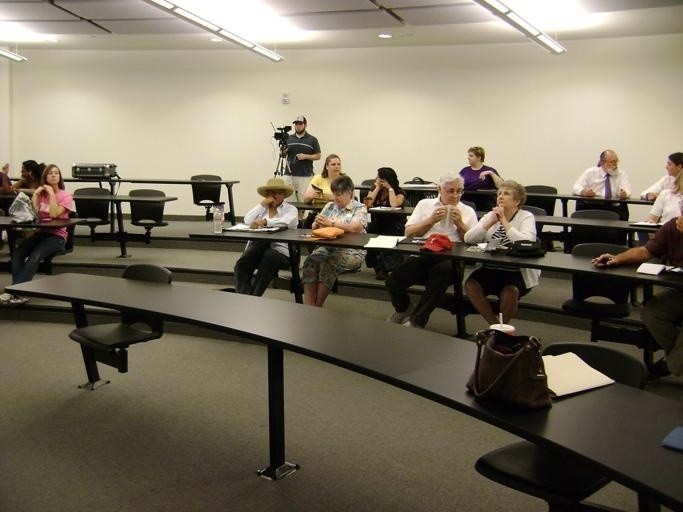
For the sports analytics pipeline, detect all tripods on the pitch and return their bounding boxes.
[266,149,304,204]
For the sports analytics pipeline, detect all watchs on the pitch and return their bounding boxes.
[331,217,335,227]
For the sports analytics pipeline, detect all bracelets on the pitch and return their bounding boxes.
[33,191,41,196]
[388,187,394,192]
[264,218,267,226]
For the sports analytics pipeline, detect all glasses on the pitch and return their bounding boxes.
[446,190,465,196]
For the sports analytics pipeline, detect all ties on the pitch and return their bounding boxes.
[604,174,612,199]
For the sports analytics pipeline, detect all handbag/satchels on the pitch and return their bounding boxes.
[466,328,554,414]
[312,227,344,240]
[7,193,41,227]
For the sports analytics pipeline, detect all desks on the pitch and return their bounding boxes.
[264,196,661,237]
[2,176,248,225]
[189,220,683,340]
[5,271,682,508]
[0,214,86,277]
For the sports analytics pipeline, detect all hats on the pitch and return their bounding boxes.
[509,240,545,258]
[256,178,295,200]
[420,233,454,253]
[293,116,308,124]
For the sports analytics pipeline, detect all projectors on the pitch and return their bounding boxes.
[72,164,117,177]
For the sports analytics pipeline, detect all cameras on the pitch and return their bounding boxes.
[270,125,293,147]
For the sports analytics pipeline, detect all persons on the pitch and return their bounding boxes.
[641,152,683,200]
[592,199,683,384]
[11,159,38,190]
[280,114,322,220]
[361,167,407,280]
[303,153,359,229]
[573,149,632,221]
[455,145,504,213]
[0,168,74,305]
[464,180,541,324]
[0,164,15,217]
[233,178,302,297]
[301,176,371,307]
[15,163,48,238]
[385,176,478,328]
[648,170,683,226]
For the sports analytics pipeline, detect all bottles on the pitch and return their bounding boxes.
[213,209,222,233]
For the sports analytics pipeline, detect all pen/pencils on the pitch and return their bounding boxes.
[601,257,612,261]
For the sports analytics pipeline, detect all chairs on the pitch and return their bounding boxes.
[566,211,617,252]
[474,342,645,503]
[67,262,171,355]
[360,178,377,202]
[73,186,112,235]
[129,188,170,240]
[186,174,227,222]
[523,186,556,216]
[521,206,545,238]
[561,242,640,318]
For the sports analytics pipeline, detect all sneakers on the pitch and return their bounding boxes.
[388,303,418,323]
[400,317,422,332]
[10,296,31,304]
[646,360,672,380]
[0,293,15,304]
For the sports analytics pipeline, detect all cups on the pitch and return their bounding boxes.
[489,323,514,335]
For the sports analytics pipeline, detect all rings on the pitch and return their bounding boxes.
[436,210,440,215]
